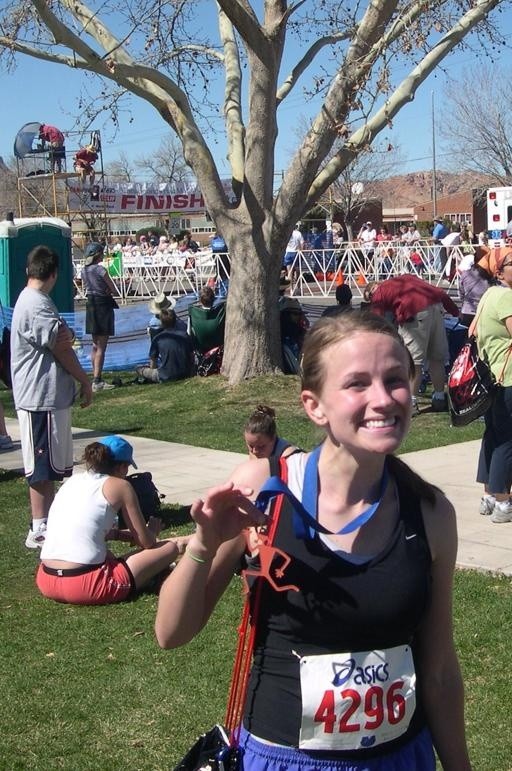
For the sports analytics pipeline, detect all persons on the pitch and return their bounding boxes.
[189,286,227,353]
[506,219,512,243]
[11,247,92,550]
[459,221,477,257]
[38,122,65,174]
[36,436,179,604]
[0,399,14,448]
[322,284,354,319]
[479,227,489,247]
[284,224,304,282]
[210,231,230,288]
[364,274,464,418]
[82,243,120,392]
[426,216,448,272]
[280,277,310,350]
[308,215,421,284]
[153,308,471,770]
[72,145,97,197]
[147,293,197,351]
[136,309,197,384]
[103,230,201,283]
[469,248,512,523]
[459,246,503,327]
[156,405,308,555]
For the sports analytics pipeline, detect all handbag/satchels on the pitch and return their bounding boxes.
[446,289,512,428]
[171,454,288,771]
[118,474,161,530]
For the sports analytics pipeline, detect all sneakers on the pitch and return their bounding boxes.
[25,522,50,550]
[492,502,512,523]
[431,393,445,411]
[479,497,496,515]
[0,435,13,448]
[92,382,115,392]
[410,399,419,416]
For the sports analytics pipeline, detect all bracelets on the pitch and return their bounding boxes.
[186,546,217,563]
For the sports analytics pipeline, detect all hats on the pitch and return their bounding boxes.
[85,243,104,266]
[433,215,443,222]
[280,297,309,314]
[148,294,176,314]
[97,435,137,470]
[366,222,372,225]
